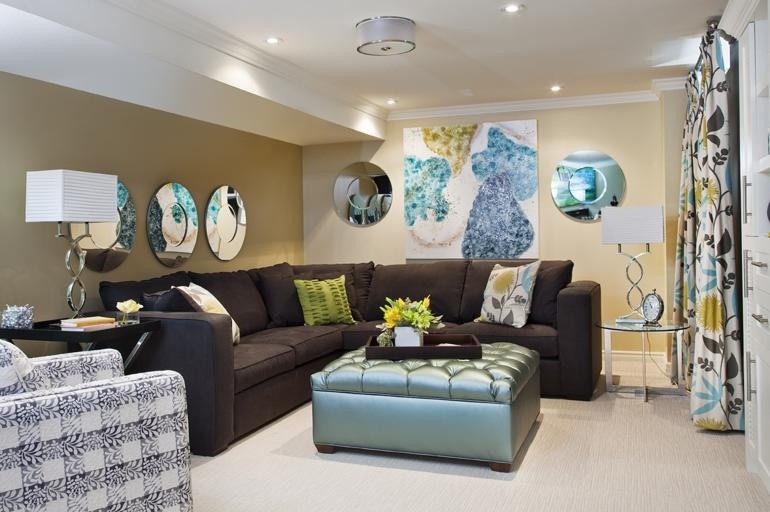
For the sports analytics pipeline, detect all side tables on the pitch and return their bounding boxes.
[602,323,689,404]
[0,315,161,371]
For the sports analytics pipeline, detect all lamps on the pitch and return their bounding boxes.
[354,15,417,56]
[26,170,118,325]
[602,203,665,323]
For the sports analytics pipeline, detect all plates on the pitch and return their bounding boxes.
[365,334,483,361]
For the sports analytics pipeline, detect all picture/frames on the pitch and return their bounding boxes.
[403,119,541,261]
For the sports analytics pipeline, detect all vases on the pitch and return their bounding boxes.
[394,326,425,346]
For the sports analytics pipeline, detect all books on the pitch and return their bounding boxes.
[61,316,116,327]
[61,322,117,332]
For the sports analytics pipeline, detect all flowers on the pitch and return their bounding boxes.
[376,297,439,347]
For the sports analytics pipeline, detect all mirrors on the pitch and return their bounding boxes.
[550,149,627,223]
[68,172,136,272]
[331,161,394,229]
[204,184,248,262]
[146,181,199,269]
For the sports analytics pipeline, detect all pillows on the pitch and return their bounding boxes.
[474,260,541,326]
[294,275,356,326]
[256,263,306,325]
[295,264,363,320]
[175,283,240,348]
[142,290,189,314]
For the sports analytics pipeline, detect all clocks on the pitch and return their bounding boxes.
[642,288,663,327]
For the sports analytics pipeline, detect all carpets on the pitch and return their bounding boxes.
[188,357,770,510]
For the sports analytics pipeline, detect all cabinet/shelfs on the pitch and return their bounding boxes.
[738,21,770,486]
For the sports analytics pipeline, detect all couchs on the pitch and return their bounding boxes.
[0,339,195,512]
[368,260,600,400]
[309,344,541,473]
[99,260,372,458]
